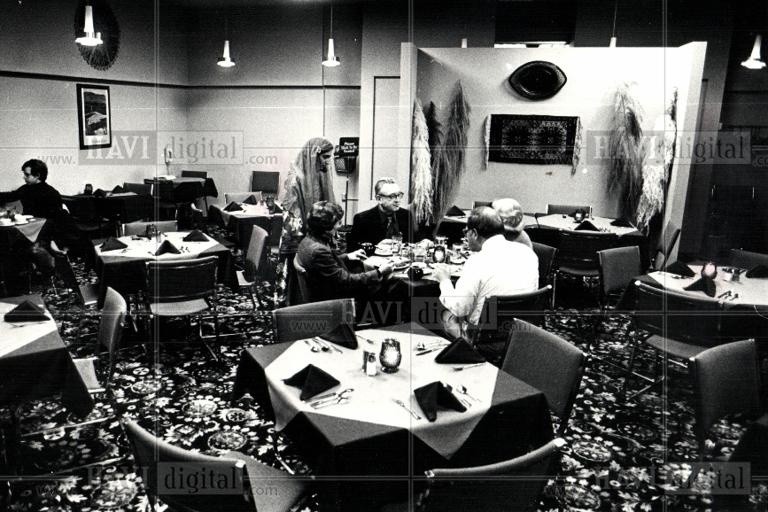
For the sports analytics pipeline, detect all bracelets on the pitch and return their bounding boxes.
[377,270,382,279]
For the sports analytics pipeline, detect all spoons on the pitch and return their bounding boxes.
[309,387,355,410]
[313,339,330,351]
[416,339,448,350]
[357,334,375,345]
[455,383,477,402]
[451,362,485,371]
[303,339,319,353]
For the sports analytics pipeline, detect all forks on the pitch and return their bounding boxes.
[718,290,742,301]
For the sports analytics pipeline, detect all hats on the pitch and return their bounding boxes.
[492,198,526,232]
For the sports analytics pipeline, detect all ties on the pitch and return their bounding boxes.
[386,216,396,238]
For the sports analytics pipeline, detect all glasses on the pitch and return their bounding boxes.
[24,172,31,177]
[378,192,404,200]
[462,228,471,237]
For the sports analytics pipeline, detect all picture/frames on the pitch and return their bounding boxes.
[73,82,114,151]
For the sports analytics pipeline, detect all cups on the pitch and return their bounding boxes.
[14,216,27,222]
[574,208,584,222]
[378,337,402,375]
[1,218,11,225]
[700,261,719,280]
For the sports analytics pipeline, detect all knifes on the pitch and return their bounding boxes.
[390,397,421,420]
[416,347,443,356]
[330,344,344,354]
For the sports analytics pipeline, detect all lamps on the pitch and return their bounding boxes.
[320,1,343,69]
[215,1,239,70]
[738,1,768,74]
[74,2,103,55]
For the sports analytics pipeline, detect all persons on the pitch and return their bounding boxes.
[490,197,533,250]
[278,137,334,294]
[433,206,540,350]
[348,178,411,252]
[1,160,63,239]
[296,201,395,301]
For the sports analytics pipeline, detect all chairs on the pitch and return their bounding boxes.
[0,170,767,511]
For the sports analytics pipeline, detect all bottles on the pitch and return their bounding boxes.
[722,266,743,283]
[361,348,377,377]
[146,224,163,246]
[588,205,593,220]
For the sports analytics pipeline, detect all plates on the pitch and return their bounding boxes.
[15,221,29,225]
[372,240,470,279]
[1,221,16,227]
